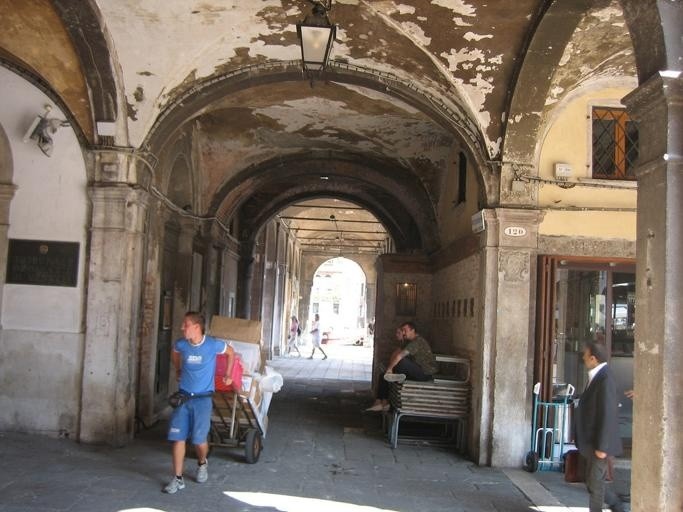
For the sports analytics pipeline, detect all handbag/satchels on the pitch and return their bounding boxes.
[296,326,302,336]
[169,392,186,408]
[565,448,586,483]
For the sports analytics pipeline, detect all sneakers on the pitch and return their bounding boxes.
[322,355,327,360]
[306,357,313,359]
[383,373,406,383]
[365,405,384,411]
[196,460,208,484]
[162,476,185,495]
[383,404,390,412]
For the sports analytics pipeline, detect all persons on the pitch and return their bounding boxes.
[574,340,629,512]
[305,313,328,360]
[383,321,441,384]
[283,315,301,359]
[161,311,236,494]
[363,325,410,412]
[622,389,633,401]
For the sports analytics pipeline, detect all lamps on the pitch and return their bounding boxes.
[294,0,341,74]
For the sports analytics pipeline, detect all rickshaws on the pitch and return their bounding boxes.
[526,382,576,474]
[167,379,264,464]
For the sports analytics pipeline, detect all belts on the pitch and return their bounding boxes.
[176,390,215,398]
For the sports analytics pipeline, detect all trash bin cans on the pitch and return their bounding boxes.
[553,399,574,444]
